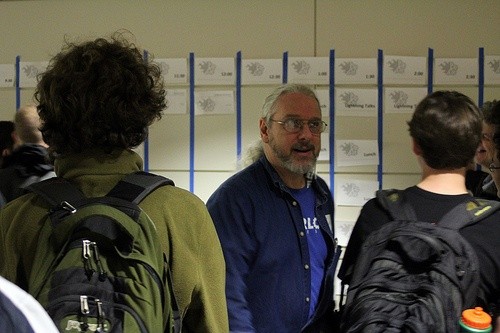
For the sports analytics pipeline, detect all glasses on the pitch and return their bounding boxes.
[270,117,328,134]
[489,163,500,172]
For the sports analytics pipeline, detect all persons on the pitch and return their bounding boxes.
[339,90,500,333]
[0,36,229,333]
[206,84,342,333]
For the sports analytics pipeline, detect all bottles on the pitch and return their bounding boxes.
[459,306,492,333]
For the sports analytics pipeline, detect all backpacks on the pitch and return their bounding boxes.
[339,189,500,333]
[25,171,182,333]
